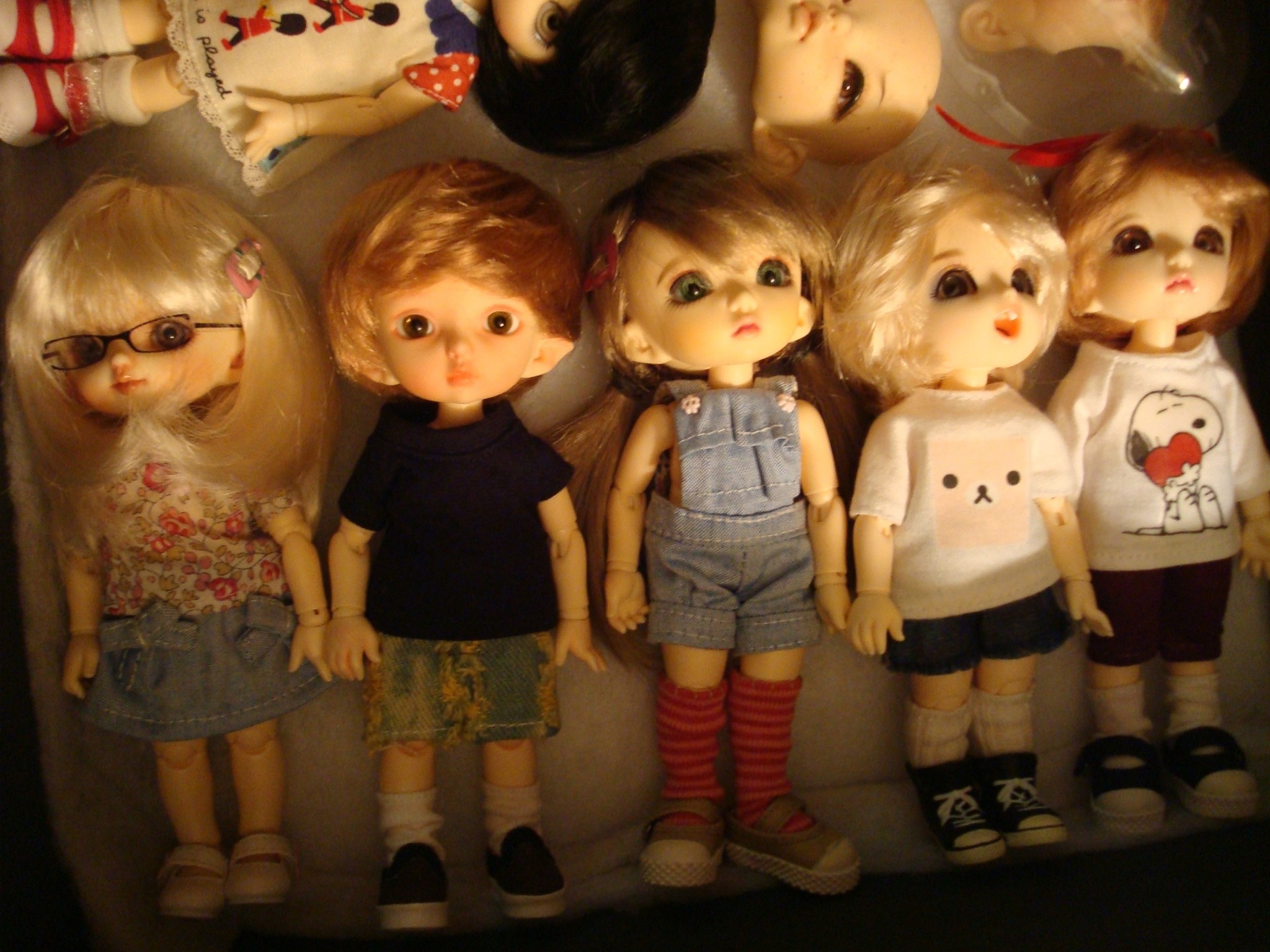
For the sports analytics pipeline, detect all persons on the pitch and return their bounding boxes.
[320,157,608,927]
[6,170,331,921]
[959,0,1207,95]
[538,147,869,893]
[753,0,942,174]
[1040,121,1270,835]
[820,143,1114,860]
[0,0,716,194]
